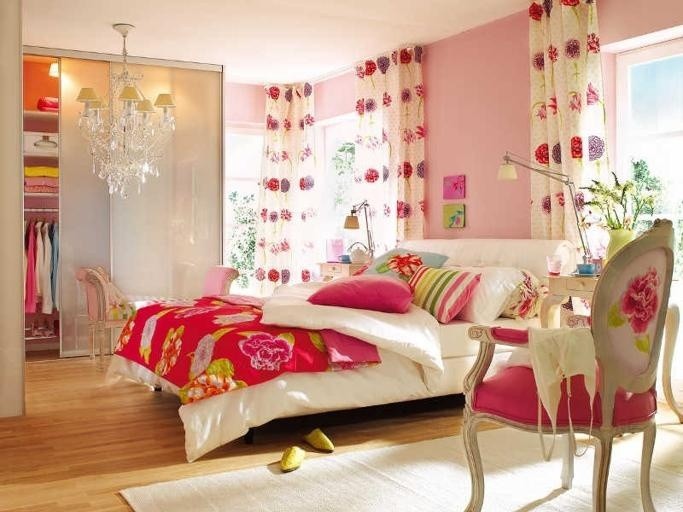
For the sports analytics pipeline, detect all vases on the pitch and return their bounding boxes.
[606,230,633,263]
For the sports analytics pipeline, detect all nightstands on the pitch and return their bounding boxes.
[540,277,683,426]
[318,263,365,281]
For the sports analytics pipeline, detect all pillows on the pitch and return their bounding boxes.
[361,247,450,282]
[501,269,540,320]
[307,275,413,313]
[441,266,525,324]
[408,265,482,323]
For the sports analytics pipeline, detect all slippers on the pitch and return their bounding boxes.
[280,446,304,472]
[303,428,334,451]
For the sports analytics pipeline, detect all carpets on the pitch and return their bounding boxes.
[117,411,683,512]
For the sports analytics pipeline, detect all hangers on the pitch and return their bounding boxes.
[24,208,57,226]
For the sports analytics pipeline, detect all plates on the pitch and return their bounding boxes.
[575,274,601,277]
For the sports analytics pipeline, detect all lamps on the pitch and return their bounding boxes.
[496,150,594,276]
[344,200,375,264]
[77,24,177,199]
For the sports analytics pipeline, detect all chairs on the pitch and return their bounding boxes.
[76,267,142,363]
[461,218,675,512]
[204,265,239,299]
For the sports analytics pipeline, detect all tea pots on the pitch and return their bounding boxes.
[347,241,369,264]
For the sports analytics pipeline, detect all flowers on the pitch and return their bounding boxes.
[578,158,664,230]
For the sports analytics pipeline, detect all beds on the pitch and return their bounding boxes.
[106,238,577,463]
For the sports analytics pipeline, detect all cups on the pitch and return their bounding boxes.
[577,263,596,274]
[547,255,562,276]
[338,255,349,262]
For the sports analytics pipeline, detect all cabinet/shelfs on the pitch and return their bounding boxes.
[22,44,226,358]
